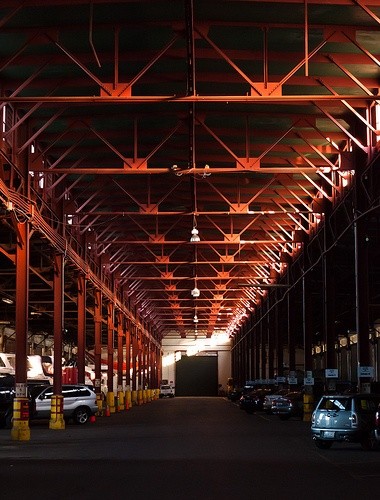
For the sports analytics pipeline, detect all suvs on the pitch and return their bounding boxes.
[30,385,99,425]
[159,385,175,398]
[310,393,380,449]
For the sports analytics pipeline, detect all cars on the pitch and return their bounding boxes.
[0,373,36,429]
[240,388,274,414]
[101,384,108,401]
[271,392,303,419]
[263,389,298,413]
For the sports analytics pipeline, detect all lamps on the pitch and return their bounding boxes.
[192,288,200,297]
[190,227,200,242]
